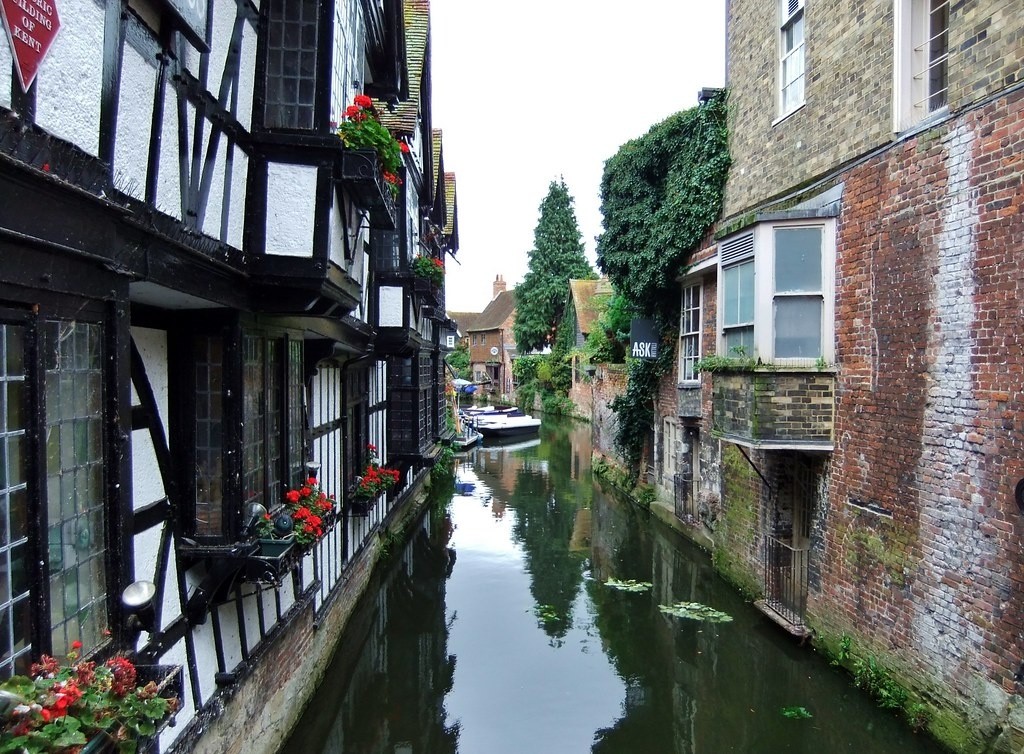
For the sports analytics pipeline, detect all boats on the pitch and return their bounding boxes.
[463,406,542,439]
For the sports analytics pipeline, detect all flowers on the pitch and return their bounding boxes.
[0,641,178,754]
[353,443,400,499]
[338,94,410,194]
[411,254,446,289]
[256,477,338,545]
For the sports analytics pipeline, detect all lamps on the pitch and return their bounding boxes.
[236,501,267,545]
[122,580,161,634]
[306,462,322,478]
